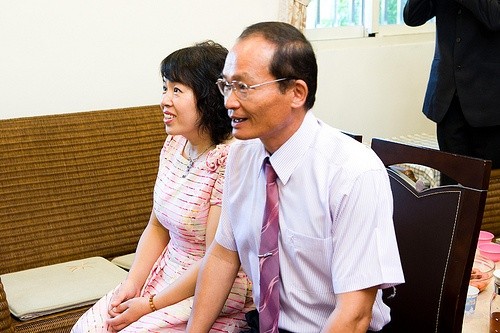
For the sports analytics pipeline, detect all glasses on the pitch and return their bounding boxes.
[215,78,297,100]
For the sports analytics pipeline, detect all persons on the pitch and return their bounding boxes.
[71,40,256,333]
[402,0,500,170]
[186,22,406,333]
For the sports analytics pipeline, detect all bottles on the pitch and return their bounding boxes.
[488,269,500,333]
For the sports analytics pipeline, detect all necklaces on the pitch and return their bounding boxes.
[181,142,214,178]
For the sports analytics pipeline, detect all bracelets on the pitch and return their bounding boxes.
[149,294,157,311]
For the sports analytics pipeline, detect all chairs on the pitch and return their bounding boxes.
[367,137,491,333]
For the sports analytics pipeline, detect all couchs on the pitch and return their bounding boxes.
[0,105,169,333]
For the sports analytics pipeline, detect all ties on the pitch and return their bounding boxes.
[258,163,280,333]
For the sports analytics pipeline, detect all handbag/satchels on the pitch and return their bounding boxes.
[388,163,442,193]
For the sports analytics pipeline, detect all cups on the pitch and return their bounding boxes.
[464,286,480,320]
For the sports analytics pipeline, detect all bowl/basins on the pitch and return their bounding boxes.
[470,254,496,293]
[478,230,494,245]
[478,243,500,262]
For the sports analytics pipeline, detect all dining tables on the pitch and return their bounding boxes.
[462,241,500,333]
